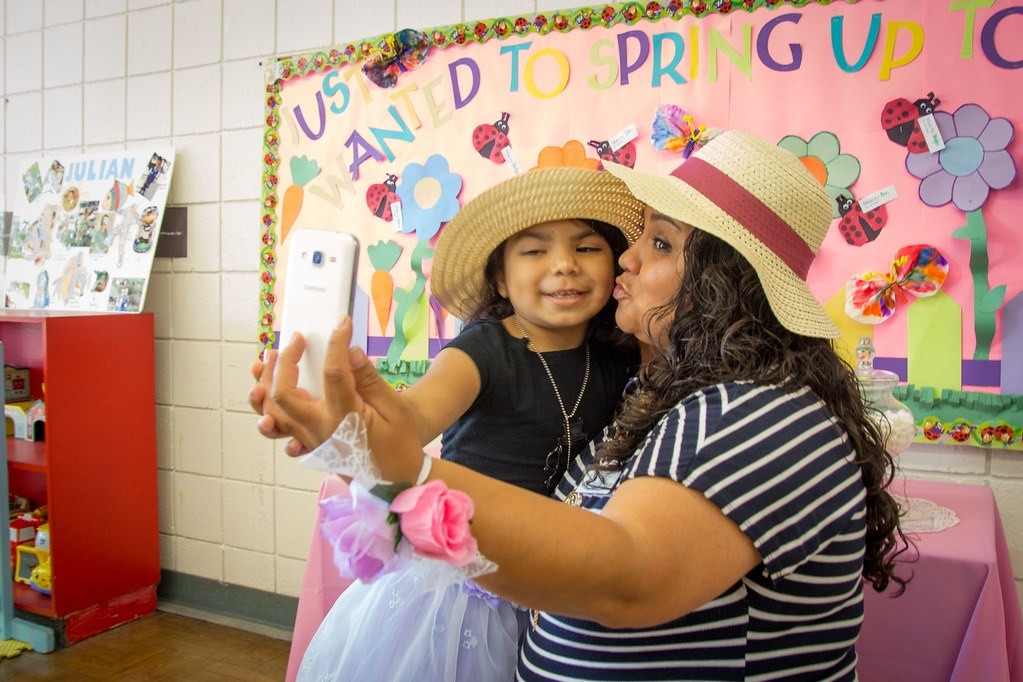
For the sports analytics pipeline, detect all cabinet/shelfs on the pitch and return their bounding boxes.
[0,308,160,648]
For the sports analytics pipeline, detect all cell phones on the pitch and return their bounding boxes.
[277,228,361,397]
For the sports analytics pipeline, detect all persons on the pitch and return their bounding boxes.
[67,189,74,205]
[250,164,647,682]
[113,296,130,312]
[139,158,163,194]
[260,127,921,682]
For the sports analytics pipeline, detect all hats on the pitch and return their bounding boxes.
[430,167,644,323]
[600,129,843,340]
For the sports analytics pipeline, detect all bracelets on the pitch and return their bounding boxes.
[302,412,498,612]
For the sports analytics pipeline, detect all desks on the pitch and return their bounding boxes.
[286,473,1023,682]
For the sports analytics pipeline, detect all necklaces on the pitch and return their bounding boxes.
[515,318,592,470]
